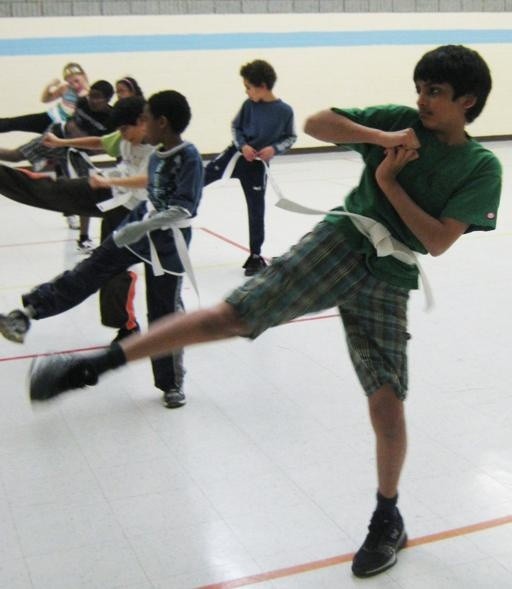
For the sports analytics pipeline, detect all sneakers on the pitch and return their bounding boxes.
[75,238,99,253]
[111,326,141,348]
[351,506,408,578]
[162,387,187,409]
[243,256,266,276]
[0,310,30,343]
[29,352,98,402]
[67,213,81,228]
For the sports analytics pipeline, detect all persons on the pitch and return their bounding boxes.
[115,74,142,102]
[0,88,205,409]
[205,57,300,279]
[26,41,503,580]
[0,62,90,229]
[0,96,163,347]
[0,80,116,255]
